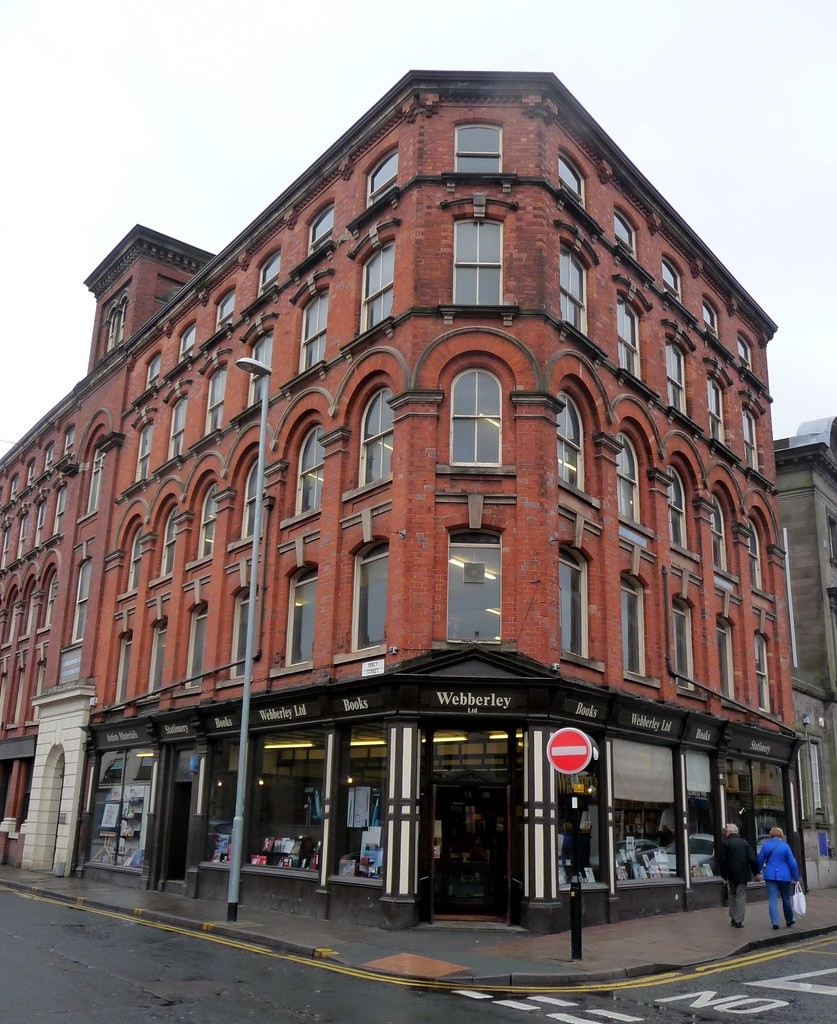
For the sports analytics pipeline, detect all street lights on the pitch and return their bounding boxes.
[226,357,274,920]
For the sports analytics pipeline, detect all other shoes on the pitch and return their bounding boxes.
[773,925,780,930]
[786,920,795,927]
[730,918,744,928]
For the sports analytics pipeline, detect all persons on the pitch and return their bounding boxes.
[720,824,762,928]
[757,827,799,929]
[660,825,673,844]
[560,822,572,863]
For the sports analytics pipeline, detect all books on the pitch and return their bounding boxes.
[617,836,669,881]
[250,836,301,866]
[210,834,231,864]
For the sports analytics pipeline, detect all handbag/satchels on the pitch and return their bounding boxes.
[791,882,806,918]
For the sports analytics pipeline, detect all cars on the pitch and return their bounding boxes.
[587,840,661,877]
[645,833,717,878]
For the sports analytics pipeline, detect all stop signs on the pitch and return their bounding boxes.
[547,728,591,775]
